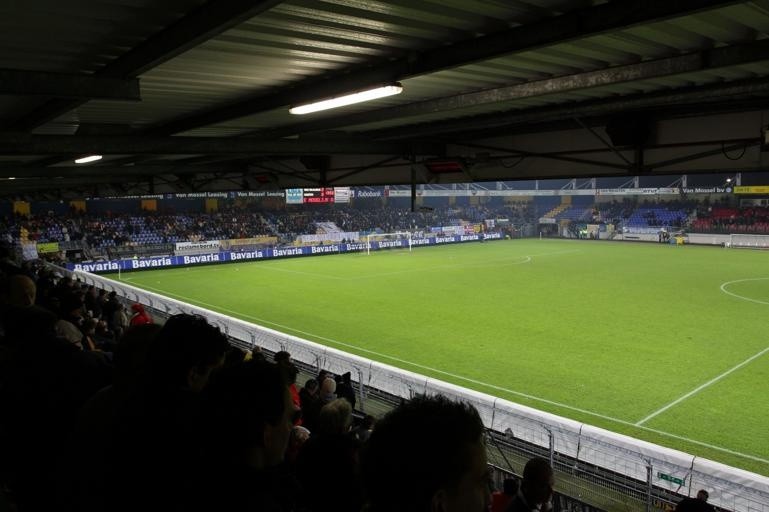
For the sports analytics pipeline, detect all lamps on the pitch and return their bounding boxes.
[75,154,104,165]
[287,80,404,116]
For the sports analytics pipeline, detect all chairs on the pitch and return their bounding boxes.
[1,198,510,267]
[521,201,766,232]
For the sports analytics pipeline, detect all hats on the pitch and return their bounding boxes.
[323,378,336,392]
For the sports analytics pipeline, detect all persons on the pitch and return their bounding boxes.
[1,195,272,260]
[645,212,660,226]
[712,197,767,228]
[643,198,715,218]
[589,196,636,229]
[0,255,558,512]
[466,197,530,233]
[269,203,459,246]
[576,228,595,238]
